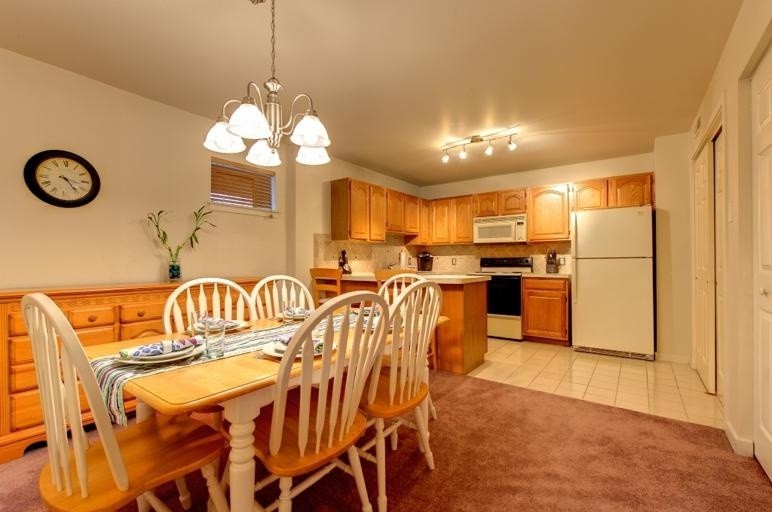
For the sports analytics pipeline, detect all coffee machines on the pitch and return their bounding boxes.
[416,252,434,274]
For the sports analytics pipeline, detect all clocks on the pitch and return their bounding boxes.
[23,149,102,209]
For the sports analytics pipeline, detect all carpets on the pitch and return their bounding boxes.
[0,355,772,511]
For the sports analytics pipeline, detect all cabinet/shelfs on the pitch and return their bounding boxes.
[435,281,490,376]
[526,182,571,243]
[330,177,386,243]
[429,195,473,245]
[474,186,526,217]
[520,273,572,348]
[405,194,429,246]
[386,189,420,236]
[571,173,653,212]
[1,284,121,464]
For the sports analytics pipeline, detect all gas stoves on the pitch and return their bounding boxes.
[465,266,532,277]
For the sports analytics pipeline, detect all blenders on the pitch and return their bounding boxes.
[545,248,559,273]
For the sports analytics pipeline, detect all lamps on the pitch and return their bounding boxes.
[201,0,334,171]
[441,129,518,164]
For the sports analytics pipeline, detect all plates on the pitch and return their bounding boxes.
[130,344,197,360]
[116,343,206,365]
[186,298,404,358]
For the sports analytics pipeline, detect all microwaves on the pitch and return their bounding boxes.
[472,213,528,244]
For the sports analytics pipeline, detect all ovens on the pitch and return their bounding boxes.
[488,274,524,341]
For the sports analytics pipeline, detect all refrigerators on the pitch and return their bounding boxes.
[568,204,656,361]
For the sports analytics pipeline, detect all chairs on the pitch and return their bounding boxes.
[22,274,452,512]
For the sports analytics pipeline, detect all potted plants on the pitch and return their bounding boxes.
[146,202,220,282]
